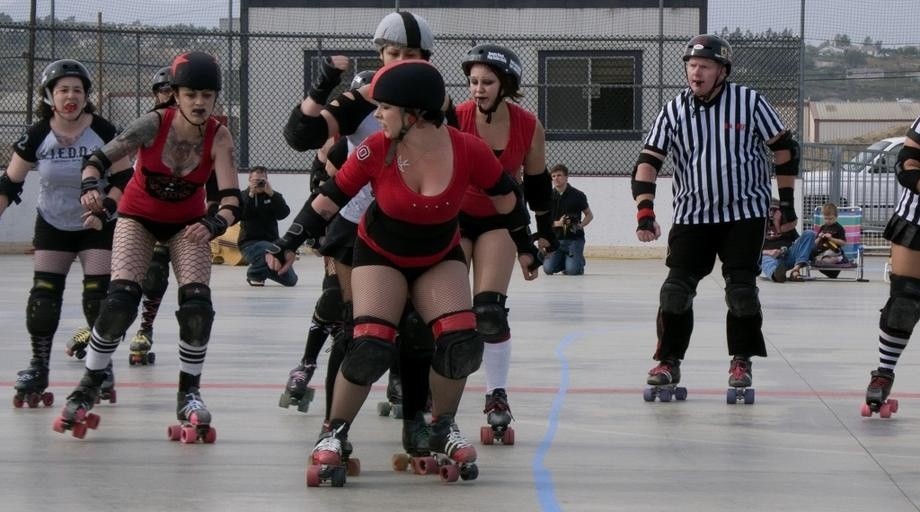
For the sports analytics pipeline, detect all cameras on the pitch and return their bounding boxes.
[767,215,774,226]
[253,179,266,189]
[563,214,579,228]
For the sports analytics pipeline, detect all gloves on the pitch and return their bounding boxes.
[306,55,343,107]
[535,211,560,253]
[199,212,228,241]
[79,176,101,203]
[775,187,798,225]
[508,224,545,272]
[264,223,310,266]
[89,198,116,229]
[636,199,656,235]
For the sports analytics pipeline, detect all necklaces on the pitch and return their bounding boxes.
[398,148,427,173]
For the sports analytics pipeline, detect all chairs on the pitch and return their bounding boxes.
[805,204,864,282]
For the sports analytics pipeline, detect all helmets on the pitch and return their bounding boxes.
[170,52,222,91]
[373,11,433,55]
[368,60,445,110]
[152,66,173,98]
[349,69,376,92]
[462,44,523,87]
[683,34,733,65]
[38,58,92,93]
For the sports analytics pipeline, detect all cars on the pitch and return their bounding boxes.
[763,137,916,232]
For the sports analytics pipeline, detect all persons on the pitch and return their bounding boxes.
[239,166,300,289]
[273,11,560,476]
[760,200,817,282]
[629,34,800,405]
[265,60,545,488]
[66,66,176,366]
[48,50,246,445]
[0,59,136,409]
[540,165,594,276]
[812,203,850,267]
[860,115,919,419]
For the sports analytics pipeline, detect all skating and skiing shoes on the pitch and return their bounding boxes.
[168,385,215,443]
[95,359,116,403]
[13,358,53,408]
[65,326,92,359]
[726,355,754,404]
[53,367,107,439]
[278,361,515,486]
[862,367,898,418]
[129,326,155,365]
[644,358,687,402]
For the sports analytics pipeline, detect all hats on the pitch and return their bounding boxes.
[768,197,781,208]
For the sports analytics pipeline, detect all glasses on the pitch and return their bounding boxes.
[158,85,173,96]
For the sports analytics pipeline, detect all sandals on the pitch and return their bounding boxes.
[790,269,805,282]
